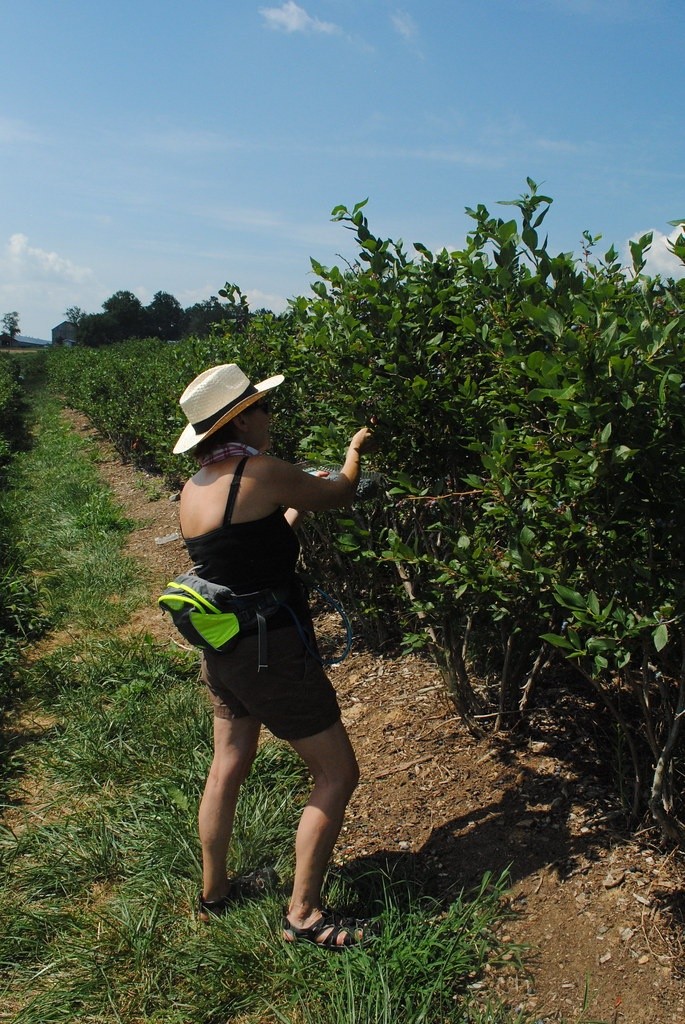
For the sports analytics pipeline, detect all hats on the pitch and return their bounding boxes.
[173,364,285,454]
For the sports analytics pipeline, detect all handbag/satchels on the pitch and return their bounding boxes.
[158,574,274,655]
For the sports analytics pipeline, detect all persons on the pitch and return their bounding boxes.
[159,363,375,948]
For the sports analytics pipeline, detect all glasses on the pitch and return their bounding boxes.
[248,403,269,414]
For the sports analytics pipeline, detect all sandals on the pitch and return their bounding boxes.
[197,865,278,925]
[281,907,374,948]
